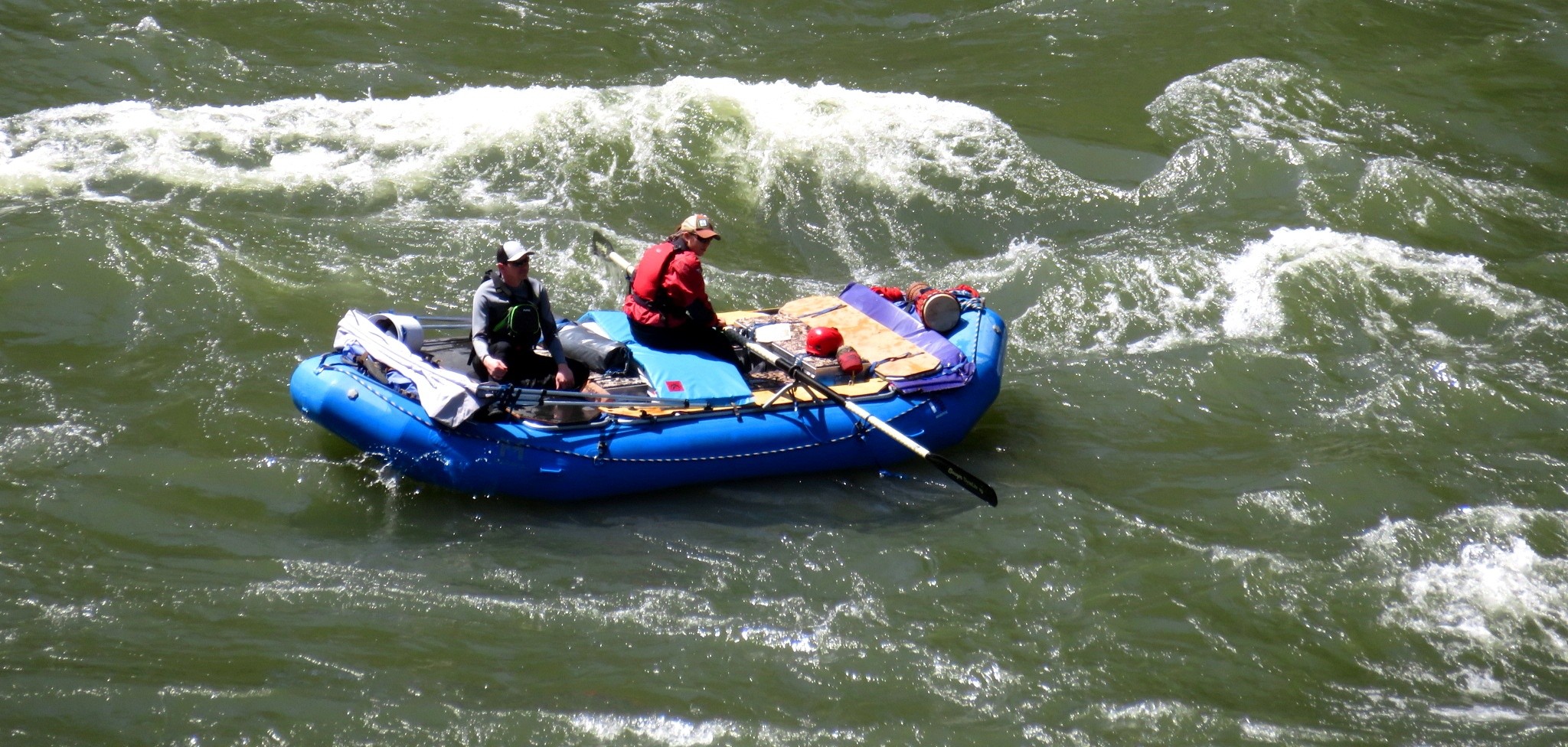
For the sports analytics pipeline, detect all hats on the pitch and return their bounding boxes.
[496,241,535,262]
[681,214,721,241]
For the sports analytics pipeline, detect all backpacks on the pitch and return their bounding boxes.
[484,270,542,351]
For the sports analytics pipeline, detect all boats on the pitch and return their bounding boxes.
[288,279,1005,505]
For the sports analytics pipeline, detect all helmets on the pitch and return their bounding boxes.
[806,326,844,356]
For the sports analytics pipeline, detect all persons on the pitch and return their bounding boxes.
[623,214,746,375]
[468,241,591,422]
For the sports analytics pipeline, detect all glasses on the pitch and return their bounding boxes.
[511,256,530,266]
[690,233,712,243]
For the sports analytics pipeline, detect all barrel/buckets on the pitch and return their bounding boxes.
[368,313,424,351]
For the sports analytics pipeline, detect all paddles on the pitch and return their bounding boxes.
[591,226,1000,507]
